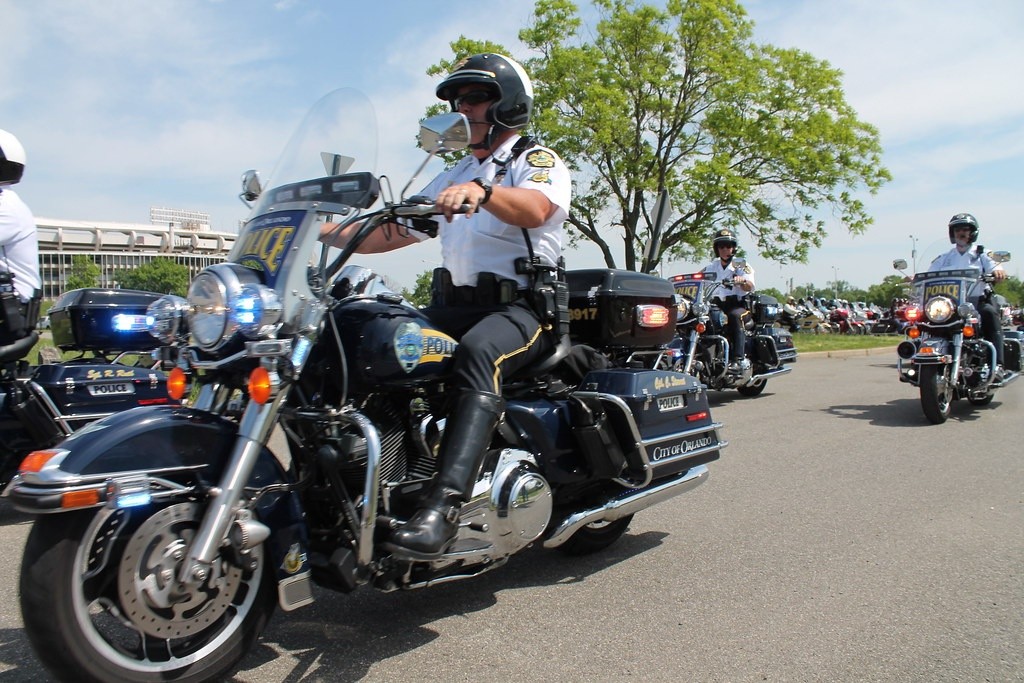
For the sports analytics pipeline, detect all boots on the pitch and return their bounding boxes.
[986,329,1004,383]
[722,328,745,375]
[384,388,506,561]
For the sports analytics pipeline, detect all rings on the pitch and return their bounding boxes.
[462,189,466,192]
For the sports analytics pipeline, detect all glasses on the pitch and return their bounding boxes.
[954,227,971,232]
[458,89,498,106]
[717,244,733,249]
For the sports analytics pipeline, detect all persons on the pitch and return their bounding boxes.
[890,299,908,332]
[317,53,571,559]
[899,213,1008,384]
[782,296,862,333]
[699,230,755,375]
[0,129,42,366]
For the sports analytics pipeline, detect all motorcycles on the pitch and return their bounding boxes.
[776,302,1024,333]
[892,250,1024,425]
[0,109,728,683]
[0,289,192,489]
[658,274,798,400]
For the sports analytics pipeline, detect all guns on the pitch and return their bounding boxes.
[984,289,994,298]
[514,256,555,286]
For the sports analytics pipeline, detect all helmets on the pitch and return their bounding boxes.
[436,53,533,128]
[786,295,868,308]
[948,212,979,243]
[712,229,739,258]
[890,297,908,304]
[0,130,25,183]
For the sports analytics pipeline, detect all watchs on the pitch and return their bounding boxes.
[471,177,492,204]
